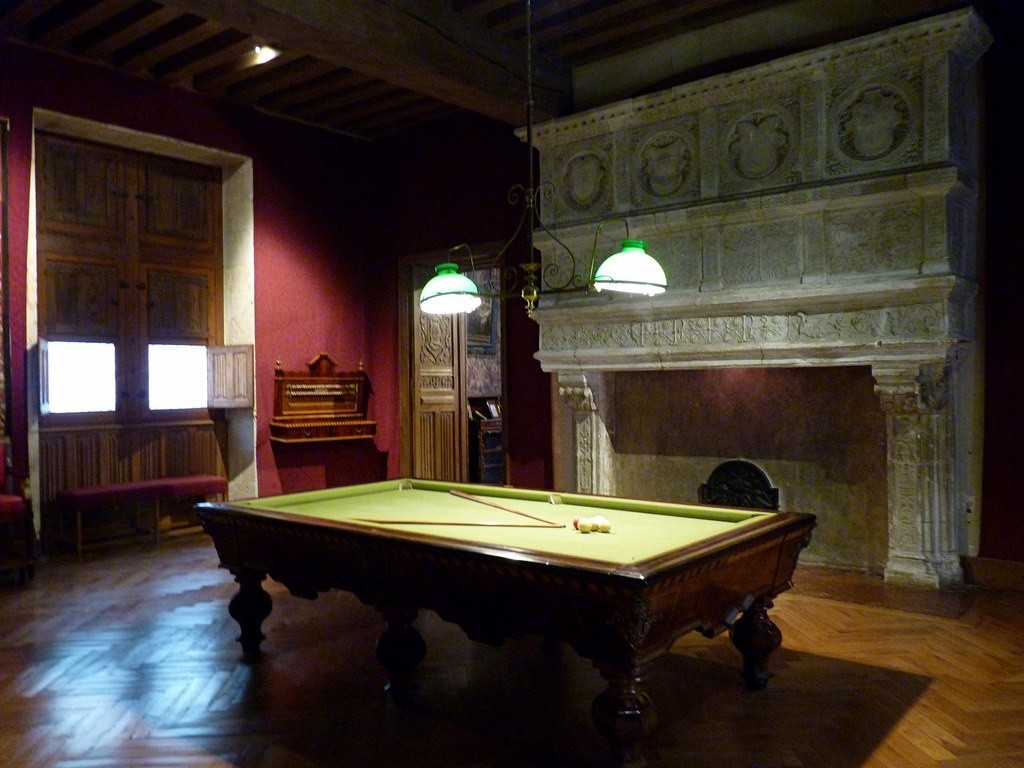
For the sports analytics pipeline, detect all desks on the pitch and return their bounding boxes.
[191,476,819,768]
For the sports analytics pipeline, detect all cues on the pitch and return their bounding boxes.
[346,489,566,529]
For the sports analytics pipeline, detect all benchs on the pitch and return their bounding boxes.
[0,494,35,579]
[54,475,230,555]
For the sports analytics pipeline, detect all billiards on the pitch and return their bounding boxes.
[572,515,612,535]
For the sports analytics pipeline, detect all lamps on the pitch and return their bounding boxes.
[415,0,670,322]
